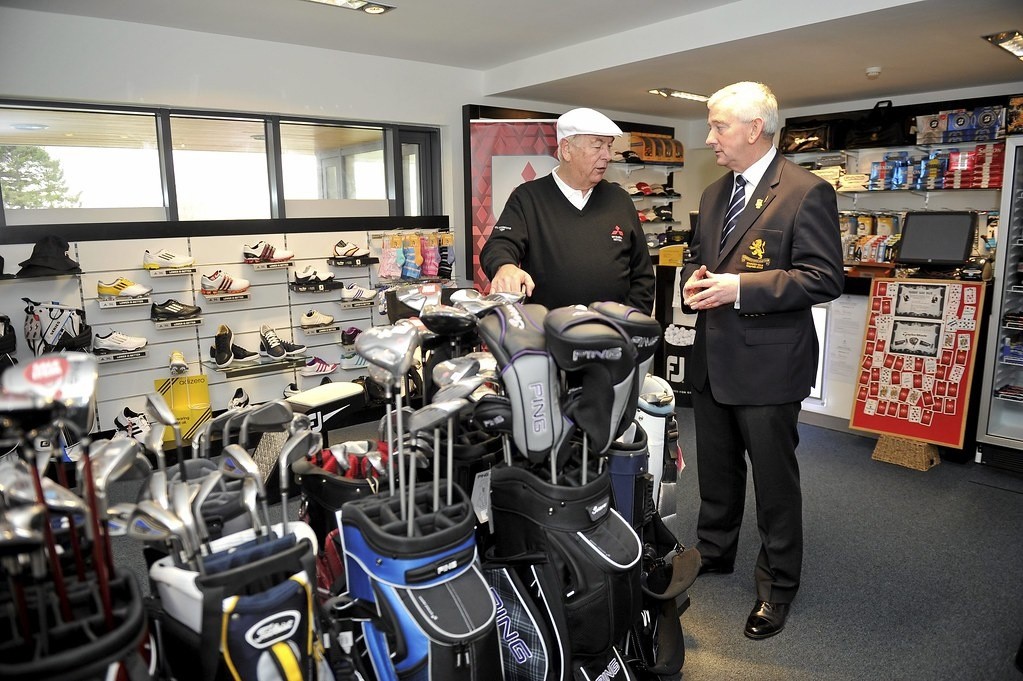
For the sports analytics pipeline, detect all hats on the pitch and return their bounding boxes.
[556,107,622,143]
[16,236,82,277]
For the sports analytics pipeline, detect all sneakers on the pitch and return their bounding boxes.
[243,240,295,265]
[294,266,335,284]
[169,352,188,373]
[201,269,251,295]
[211,344,261,362]
[259,323,287,360]
[143,248,196,271]
[300,310,336,329]
[96,277,153,301]
[216,325,234,370]
[300,328,371,391]
[341,282,378,303]
[228,387,250,409]
[93,327,147,356]
[283,383,301,398]
[258,334,309,356]
[150,297,202,321]
[111,408,166,448]
[334,242,370,258]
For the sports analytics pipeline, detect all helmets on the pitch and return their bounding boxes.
[24,302,81,359]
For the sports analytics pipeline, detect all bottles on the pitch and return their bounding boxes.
[1017,257,1023,285]
[870,234,889,262]
[842,235,856,261]
[878,234,902,262]
[1004,337,1010,357]
[849,235,866,262]
[862,235,877,262]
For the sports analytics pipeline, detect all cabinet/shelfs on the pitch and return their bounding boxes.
[612,133,684,251]
[781,93,1007,270]
[0,230,455,488]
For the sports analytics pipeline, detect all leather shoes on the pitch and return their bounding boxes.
[700,560,736,576]
[744,594,789,640]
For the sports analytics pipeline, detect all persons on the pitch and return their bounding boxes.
[479,107,657,317]
[679,82,844,639]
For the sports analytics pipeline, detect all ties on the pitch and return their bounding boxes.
[720,176,748,255]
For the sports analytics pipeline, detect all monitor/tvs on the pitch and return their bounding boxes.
[896,212,977,279]
